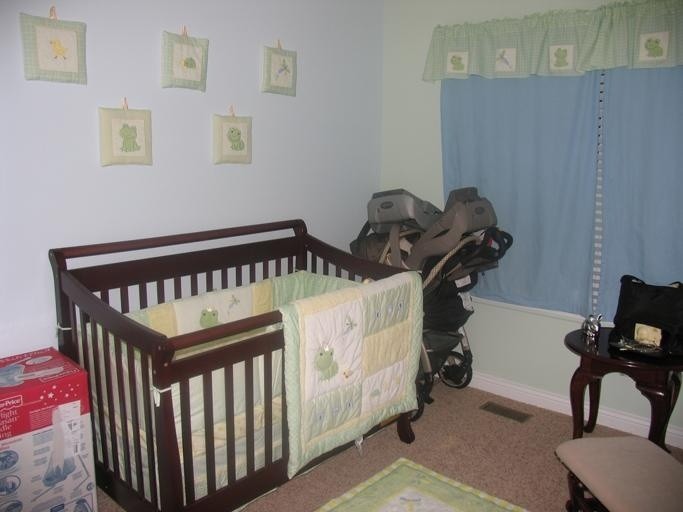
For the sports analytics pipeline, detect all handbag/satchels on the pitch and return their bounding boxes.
[350,220,424,265]
[367,189,443,236]
[614,282,682,350]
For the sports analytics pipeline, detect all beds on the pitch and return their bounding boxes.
[48,219,423,509]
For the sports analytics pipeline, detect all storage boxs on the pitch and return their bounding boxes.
[0,346,97,512]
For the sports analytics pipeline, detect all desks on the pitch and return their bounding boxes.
[565,324,683,446]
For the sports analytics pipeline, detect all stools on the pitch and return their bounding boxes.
[555,435,682,512]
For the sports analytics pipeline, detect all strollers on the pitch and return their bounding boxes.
[349,227,513,422]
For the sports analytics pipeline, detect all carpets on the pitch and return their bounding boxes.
[315,457,528,511]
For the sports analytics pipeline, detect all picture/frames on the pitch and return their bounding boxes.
[20,12,86,84]
[260,46,298,97]
[212,113,253,164]
[98,106,151,166]
[160,30,210,91]
